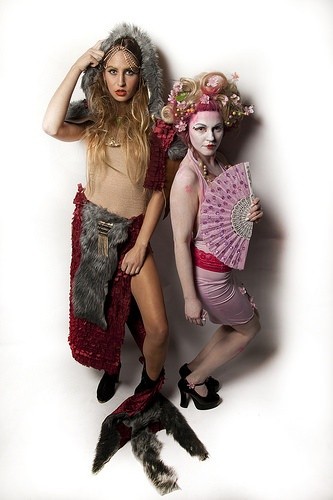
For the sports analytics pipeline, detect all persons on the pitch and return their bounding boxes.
[162,72,263,411]
[42,24,187,403]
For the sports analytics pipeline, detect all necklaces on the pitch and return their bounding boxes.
[105,118,123,147]
[197,160,230,182]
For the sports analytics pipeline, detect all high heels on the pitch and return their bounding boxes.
[178,363,221,393]
[178,377,221,411]
[135,356,165,398]
[96,361,122,404]
